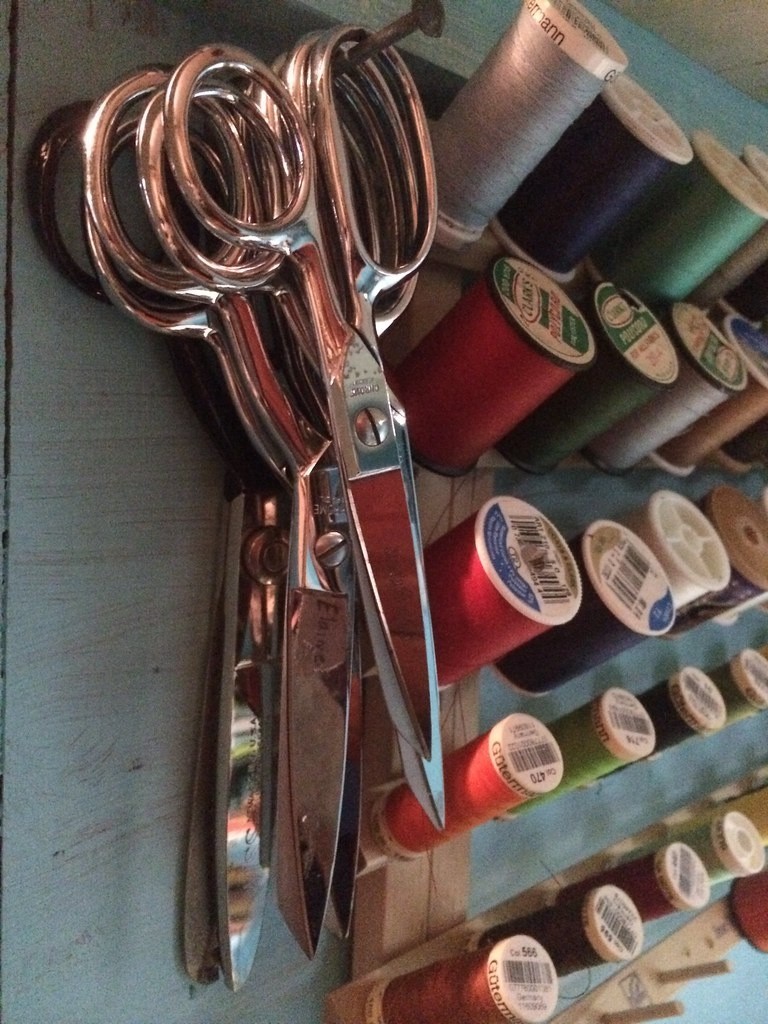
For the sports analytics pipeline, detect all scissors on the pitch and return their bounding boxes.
[24,24,448,1000]
